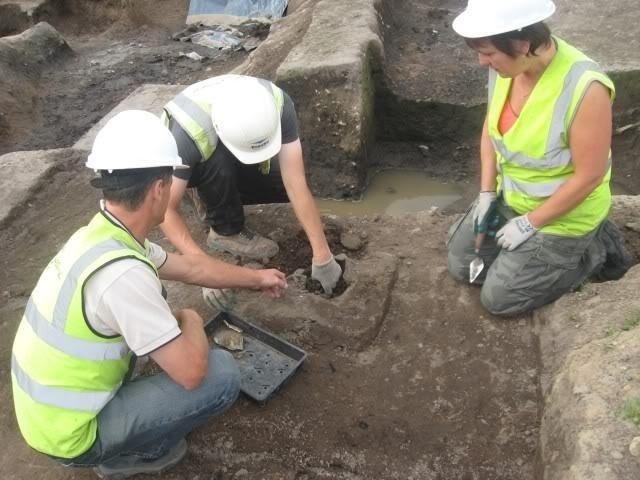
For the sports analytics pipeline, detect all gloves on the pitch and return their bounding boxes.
[493,212,540,251]
[311,253,343,295]
[471,190,500,238]
[201,285,238,313]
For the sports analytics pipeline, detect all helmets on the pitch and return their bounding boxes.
[209,75,283,165]
[84,108,191,170]
[451,0,558,39]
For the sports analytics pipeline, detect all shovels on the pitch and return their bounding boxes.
[469,223,490,284]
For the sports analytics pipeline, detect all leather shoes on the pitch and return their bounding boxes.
[207,226,279,259]
[93,438,187,480]
[599,220,631,284]
[184,187,208,224]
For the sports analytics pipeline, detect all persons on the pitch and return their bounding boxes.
[10,108,290,479]
[159,74,342,311]
[444,0,632,316]
